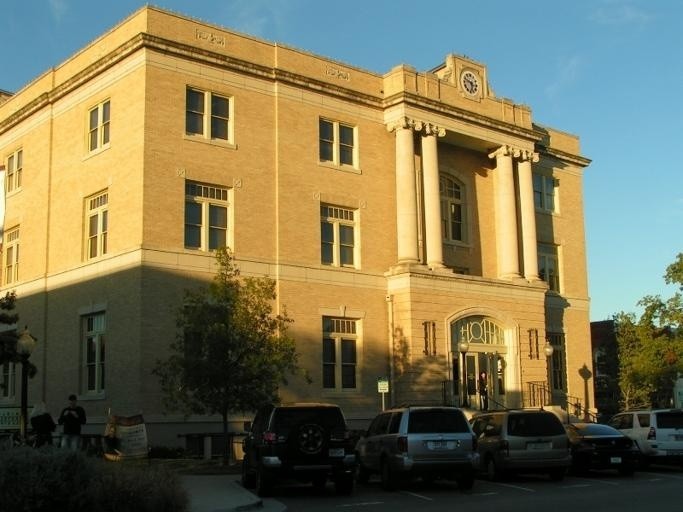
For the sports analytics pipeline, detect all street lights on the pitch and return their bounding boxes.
[457,333,470,410]
[17,321,37,447]
[541,338,554,407]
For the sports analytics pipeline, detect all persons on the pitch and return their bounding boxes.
[105,426,119,450]
[479,371,487,410]
[57,395,86,448]
[31,400,56,445]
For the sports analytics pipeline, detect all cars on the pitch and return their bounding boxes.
[567,423,637,474]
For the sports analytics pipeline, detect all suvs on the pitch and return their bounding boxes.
[466,407,573,478]
[241,402,370,498]
[607,406,681,474]
[356,406,482,488]
